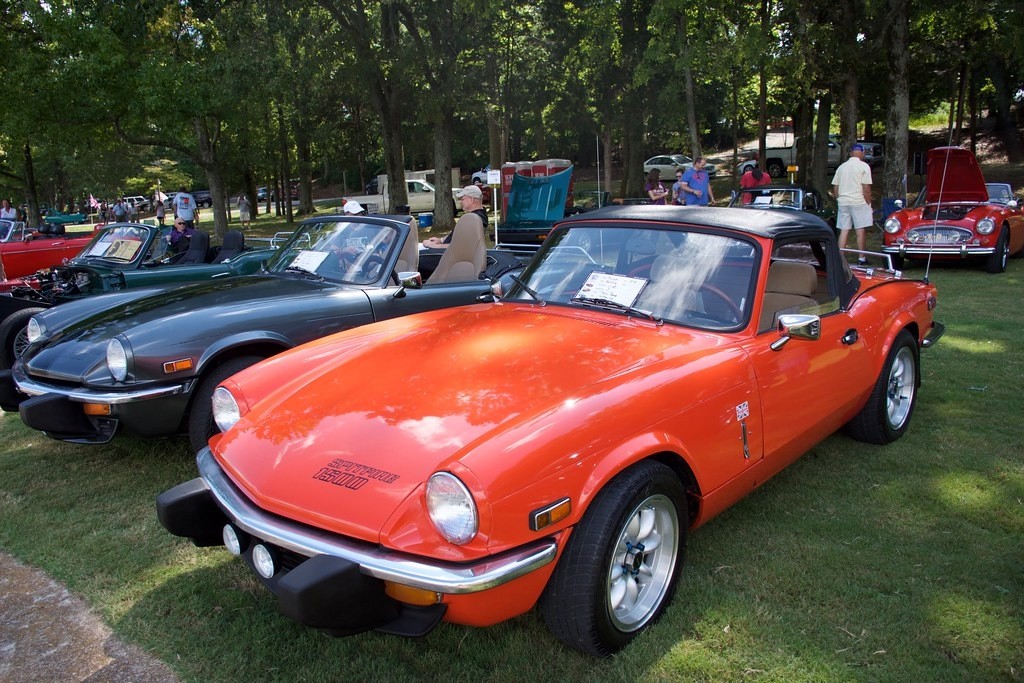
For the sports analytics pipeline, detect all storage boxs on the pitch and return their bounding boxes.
[418,212,433,226]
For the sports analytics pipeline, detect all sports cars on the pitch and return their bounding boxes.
[0,222,298,372]
[882,145,1024,273]
[490,164,611,254]
[0,218,142,296]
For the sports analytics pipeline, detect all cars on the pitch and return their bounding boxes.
[84,191,212,212]
[643,154,716,182]
[724,183,838,234]
[0,216,612,452]
[471,164,491,184]
[155,203,946,654]
[257,188,275,202]
[289,185,300,200]
[365,179,378,195]
[736,158,759,175]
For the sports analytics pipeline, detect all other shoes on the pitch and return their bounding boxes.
[857,258,875,269]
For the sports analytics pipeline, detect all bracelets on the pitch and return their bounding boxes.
[711,197,714,200]
[441,237,443,243]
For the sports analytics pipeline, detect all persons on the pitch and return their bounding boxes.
[740,162,771,206]
[645,167,668,205]
[166,217,195,244]
[39,213,46,225]
[423,184,488,249]
[95,199,110,225]
[327,200,387,276]
[110,198,140,236]
[907,193,936,212]
[64,205,79,214]
[672,168,686,206]
[171,187,200,230]
[237,195,251,230]
[154,201,165,224]
[377,228,394,247]
[0,199,26,223]
[831,145,873,267]
[679,156,716,207]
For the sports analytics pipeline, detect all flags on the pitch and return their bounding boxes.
[155,188,168,203]
[90,194,97,207]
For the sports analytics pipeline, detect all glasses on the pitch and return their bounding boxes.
[676,175,681,178]
[177,222,186,226]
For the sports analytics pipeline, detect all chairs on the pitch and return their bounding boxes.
[358,216,419,285]
[427,213,486,283]
[760,261,819,330]
[212,230,244,262]
[176,230,210,264]
[645,256,704,314]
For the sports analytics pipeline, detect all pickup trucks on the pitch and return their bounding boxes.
[765,134,842,178]
[342,179,464,217]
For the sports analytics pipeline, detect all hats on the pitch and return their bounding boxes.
[456,185,483,199]
[851,144,865,152]
